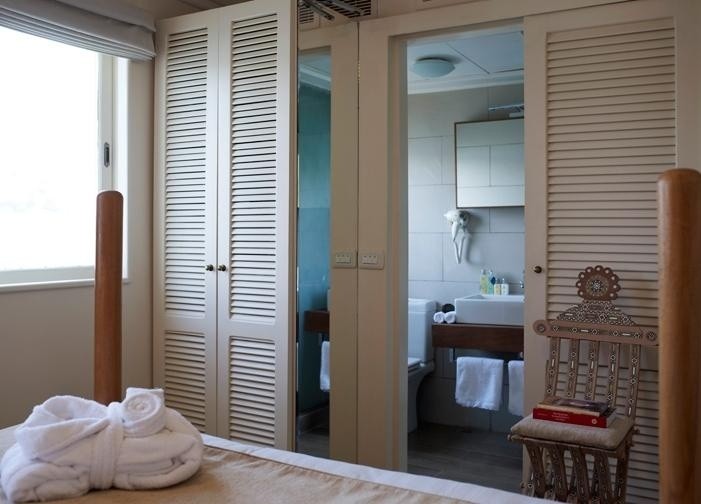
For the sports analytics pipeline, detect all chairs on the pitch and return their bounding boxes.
[508,266,658,504]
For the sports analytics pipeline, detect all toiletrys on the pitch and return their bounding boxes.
[478,269,511,294]
[479,268,510,296]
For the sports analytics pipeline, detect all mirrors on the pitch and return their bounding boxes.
[454,117,524,208]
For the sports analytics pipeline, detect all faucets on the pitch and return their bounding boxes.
[519,269,526,286]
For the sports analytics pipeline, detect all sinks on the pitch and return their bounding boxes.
[454,293,525,326]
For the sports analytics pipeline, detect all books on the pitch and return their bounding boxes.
[536,395,609,417]
[532,407,617,428]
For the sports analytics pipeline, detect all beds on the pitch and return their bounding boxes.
[31,434,562,504]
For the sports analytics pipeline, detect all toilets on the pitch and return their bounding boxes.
[407,298,437,433]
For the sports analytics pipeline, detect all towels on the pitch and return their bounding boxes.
[320,341,330,392]
[507,360,524,417]
[455,356,504,411]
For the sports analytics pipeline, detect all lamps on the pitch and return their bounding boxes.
[410,59,455,79]
